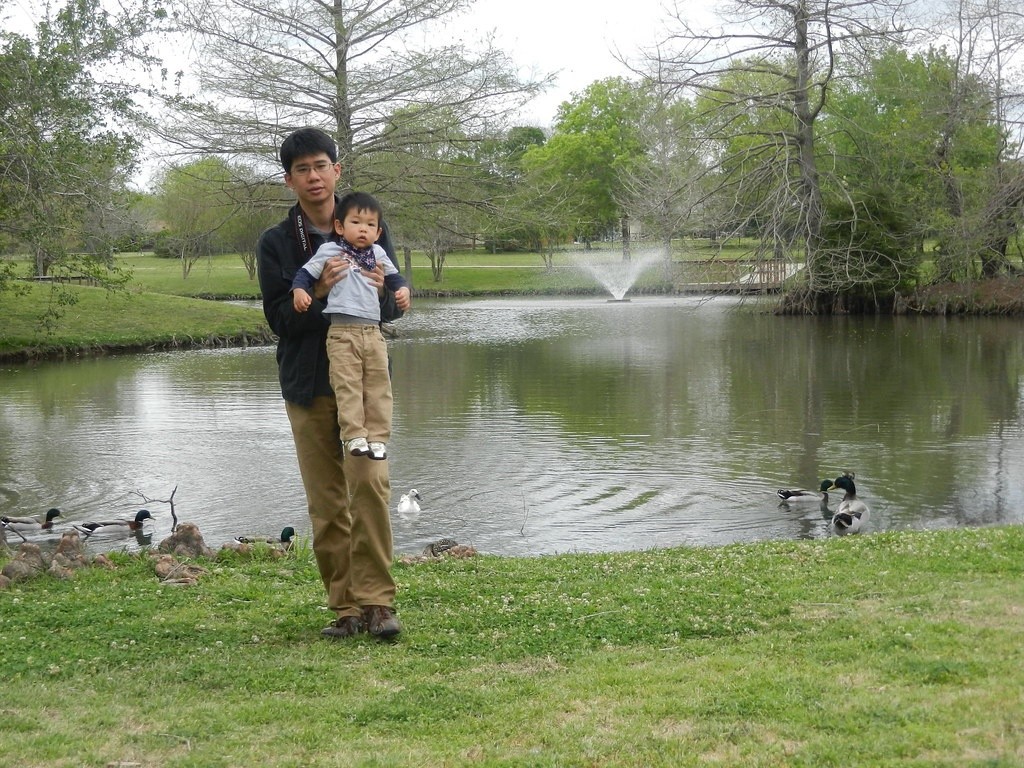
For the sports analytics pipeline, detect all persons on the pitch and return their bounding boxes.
[288,192,411,460]
[254,130,405,640]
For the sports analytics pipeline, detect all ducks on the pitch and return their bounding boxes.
[776,480,834,503]
[233,526,302,553]
[73,509,156,535]
[829,476,871,536]
[397,488,424,513]
[0,508,67,532]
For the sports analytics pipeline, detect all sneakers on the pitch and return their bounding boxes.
[362,605,400,636]
[367,441,387,460]
[344,437,370,456]
[320,616,367,637]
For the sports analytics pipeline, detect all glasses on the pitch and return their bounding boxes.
[288,163,335,175]
